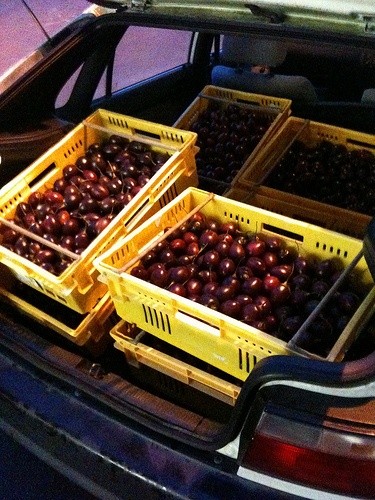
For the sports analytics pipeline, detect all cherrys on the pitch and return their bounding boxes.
[0,101,374,388]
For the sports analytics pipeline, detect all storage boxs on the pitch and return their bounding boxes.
[171,84,293,186]
[239,116,375,226]
[92,186,375,381]
[109,319,243,408]
[0,275,115,346]
[0,107,200,315]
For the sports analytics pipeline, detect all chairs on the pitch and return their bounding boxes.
[210,35,317,104]
